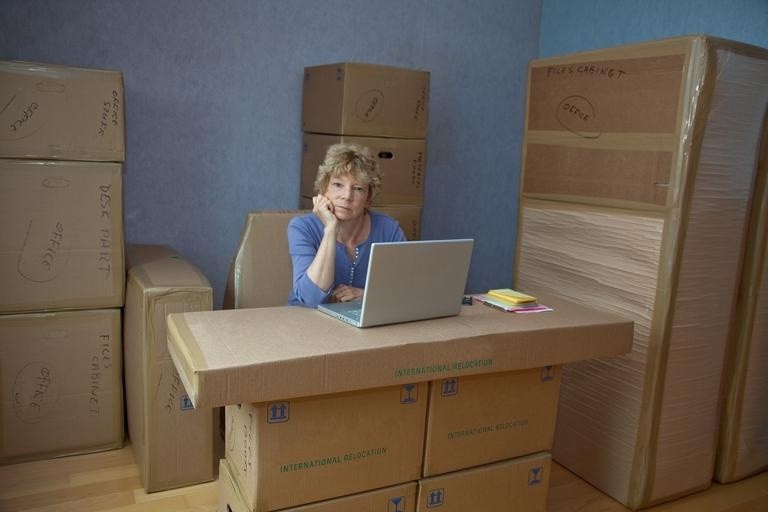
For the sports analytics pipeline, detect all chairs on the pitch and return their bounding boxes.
[224,210,309,309]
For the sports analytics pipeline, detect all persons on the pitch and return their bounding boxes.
[284,143,410,312]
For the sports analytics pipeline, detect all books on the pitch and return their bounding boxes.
[471,285,553,315]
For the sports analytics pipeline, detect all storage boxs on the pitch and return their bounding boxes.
[417,452,552,512]
[0,158,127,316]
[512,34,767,510]
[166,289,635,412]
[300,133,428,206]
[218,458,418,512]
[300,61,431,139]
[422,365,562,478]
[225,380,429,512]
[299,197,423,242]
[712,124,767,483]
[123,242,214,495]
[0,308,124,468]
[0,59,127,163]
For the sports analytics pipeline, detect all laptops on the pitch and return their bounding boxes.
[317,238,475,328]
